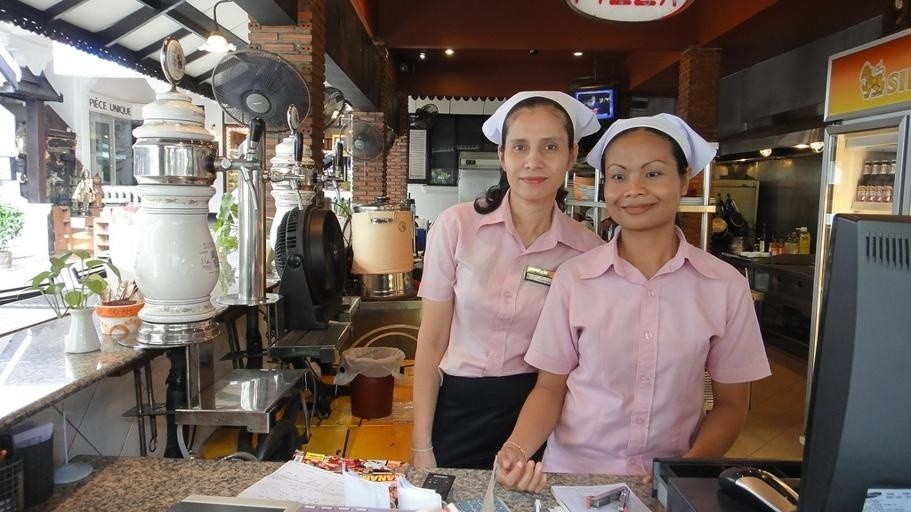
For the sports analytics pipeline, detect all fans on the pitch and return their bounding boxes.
[212,49,440,164]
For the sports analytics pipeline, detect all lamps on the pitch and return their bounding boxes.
[199,0,240,53]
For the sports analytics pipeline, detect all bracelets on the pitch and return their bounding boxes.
[503,440,530,460]
[410,444,436,452]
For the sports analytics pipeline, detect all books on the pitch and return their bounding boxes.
[342,457,402,509]
[550,482,653,512]
[293,449,344,472]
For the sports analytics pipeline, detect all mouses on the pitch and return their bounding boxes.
[718,466,799,512]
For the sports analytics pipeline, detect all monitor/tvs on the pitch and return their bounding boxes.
[798,215,911,512]
[568,80,620,126]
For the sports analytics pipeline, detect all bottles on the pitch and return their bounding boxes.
[854,160,895,201]
[759,224,811,257]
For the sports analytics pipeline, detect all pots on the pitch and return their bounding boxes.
[711,195,752,241]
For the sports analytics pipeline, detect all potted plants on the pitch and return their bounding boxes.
[0,200,26,269]
[22,246,145,357]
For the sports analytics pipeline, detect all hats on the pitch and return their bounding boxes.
[482,91,601,145]
[586,113,718,180]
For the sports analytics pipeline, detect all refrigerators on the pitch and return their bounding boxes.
[798,29,910,447]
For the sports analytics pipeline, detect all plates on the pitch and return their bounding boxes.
[679,196,716,205]
[565,176,598,203]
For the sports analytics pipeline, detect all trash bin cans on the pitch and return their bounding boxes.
[342,347,400,419]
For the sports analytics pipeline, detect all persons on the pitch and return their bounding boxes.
[597,95,610,115]
[409,90,607,472]
[491,113,774,496]
[590,95,600,114]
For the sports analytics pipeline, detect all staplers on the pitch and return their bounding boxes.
[586,485,630,512]
